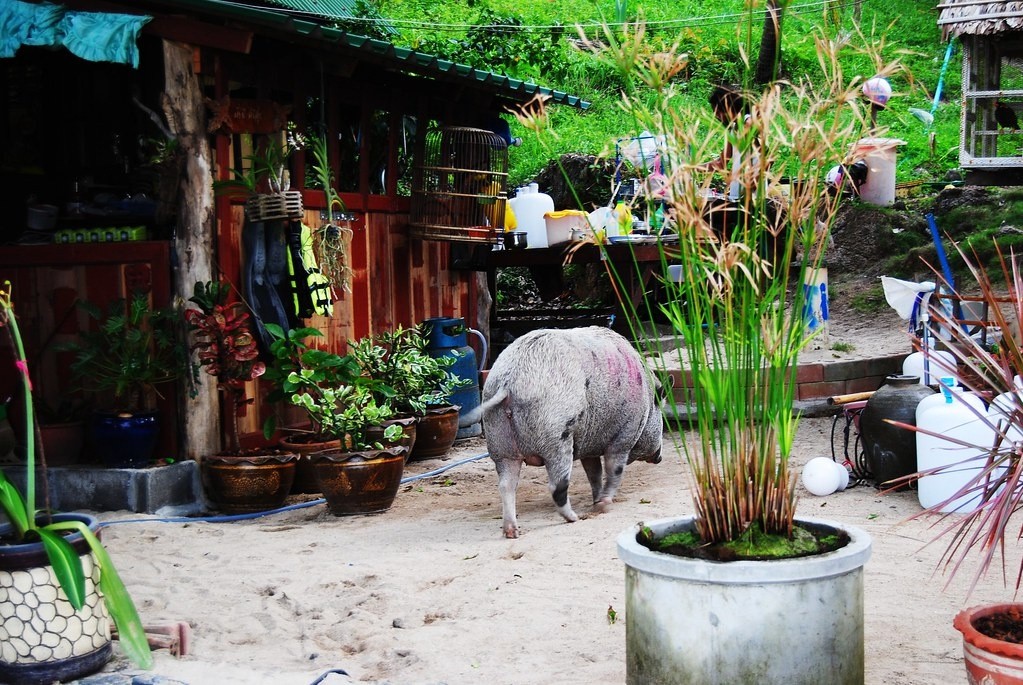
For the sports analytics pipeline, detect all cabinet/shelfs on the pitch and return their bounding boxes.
[616,135,675,235]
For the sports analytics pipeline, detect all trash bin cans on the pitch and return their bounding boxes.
[846,138,897,207]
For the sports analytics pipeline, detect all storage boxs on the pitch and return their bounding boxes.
[543,210,590,247]
[468,226,497,238]
[54,225,148,244]
[614,184,633,194]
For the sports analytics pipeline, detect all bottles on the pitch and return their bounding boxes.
[860,376,936,491]
[50,131,186,243]
[614,200,632,236]
[903,337,958,392]
[915,375,1023,514]
[507,182,554,248]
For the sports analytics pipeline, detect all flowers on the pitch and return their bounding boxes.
[0,278,156,672]
[184,280,267,455]
[211,121,309,206]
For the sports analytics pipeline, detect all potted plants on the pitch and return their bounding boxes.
[48,286,201,472]
[503,3,927,685]
[884,226,1023,685]
[263,320,474,519]
[305,131,357,293]
[31,392,88,468]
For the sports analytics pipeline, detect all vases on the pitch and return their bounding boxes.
[246,192,303,224]
[0,418,22,463]
[198,449,300,515]
[0,512,115,685]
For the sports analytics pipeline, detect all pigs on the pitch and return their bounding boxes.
[460,325,675,539]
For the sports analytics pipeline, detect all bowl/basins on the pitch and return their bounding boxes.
[504,232,527,250]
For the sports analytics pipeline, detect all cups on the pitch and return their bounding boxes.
[572,229,605,246]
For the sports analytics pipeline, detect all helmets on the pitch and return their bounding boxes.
[824,166,860,194]
[863,78,890,109]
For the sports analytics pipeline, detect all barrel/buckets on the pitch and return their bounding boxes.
[848,139,906,207]
[544,209,589,247]
